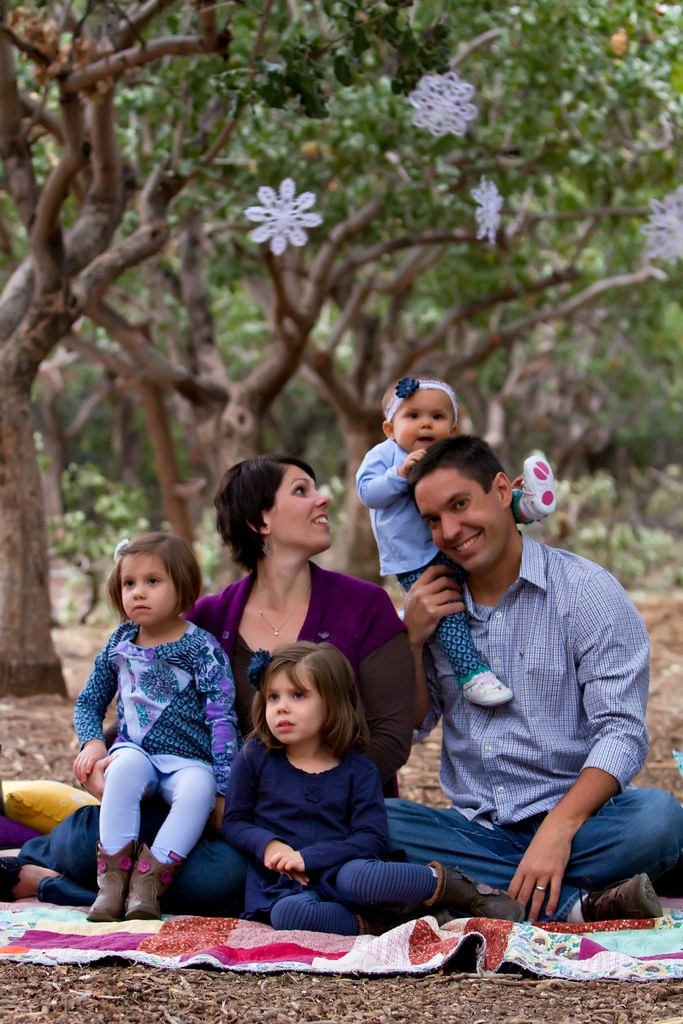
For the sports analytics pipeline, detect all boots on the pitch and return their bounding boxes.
[85,838,137,921]
[355,907,452,936]
[421,860,526,926]
[123,841,185,919]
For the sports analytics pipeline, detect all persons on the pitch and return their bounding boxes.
[0,455,417,916]
[219,641,526,938]
[68,531,241,923]
[354,373,558,709]
[384,434,683,922]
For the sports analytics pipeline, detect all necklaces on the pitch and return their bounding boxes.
[254,585,311,637]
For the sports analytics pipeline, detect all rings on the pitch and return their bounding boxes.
[535,884,546,893]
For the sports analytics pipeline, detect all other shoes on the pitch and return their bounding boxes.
[578,871,663,922]
[519,455,556,518]
[463,670,514,705]
[0,856,48,902]
